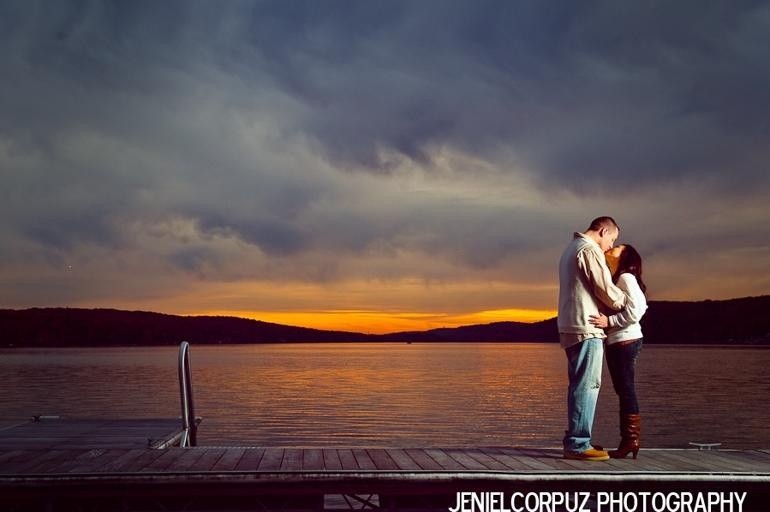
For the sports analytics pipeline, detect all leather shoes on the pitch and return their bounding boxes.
[564,442,610,460]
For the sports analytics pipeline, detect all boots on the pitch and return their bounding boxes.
[608,410,640,459]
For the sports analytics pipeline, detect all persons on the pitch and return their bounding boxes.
[558,216,620,460]
[587,243,649,459]
